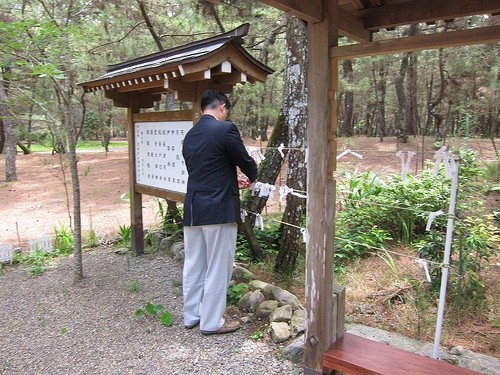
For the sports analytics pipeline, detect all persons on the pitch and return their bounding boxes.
[181,89,260,336]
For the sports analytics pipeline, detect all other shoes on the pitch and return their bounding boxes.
[185,320,200,329]
[202,321,240,335]
